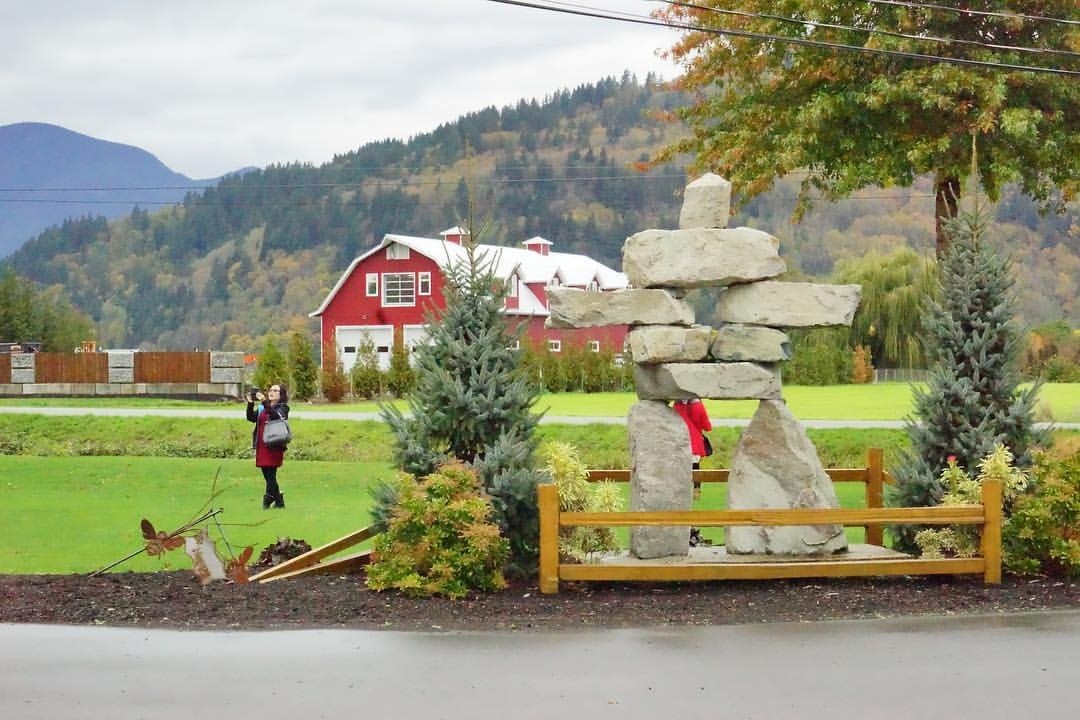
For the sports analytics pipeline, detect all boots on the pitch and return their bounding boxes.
[262,494,276,508]
[274,493,285,507]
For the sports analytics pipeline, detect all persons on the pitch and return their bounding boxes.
[246,384,290,507]
[673,398,712,542]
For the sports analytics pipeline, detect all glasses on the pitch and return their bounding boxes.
[269,389,280,392]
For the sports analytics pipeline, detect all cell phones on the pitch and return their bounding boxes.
[251,389,261,401]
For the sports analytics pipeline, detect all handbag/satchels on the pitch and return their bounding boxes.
[703,434,714,456]
[261,410,292,445]
